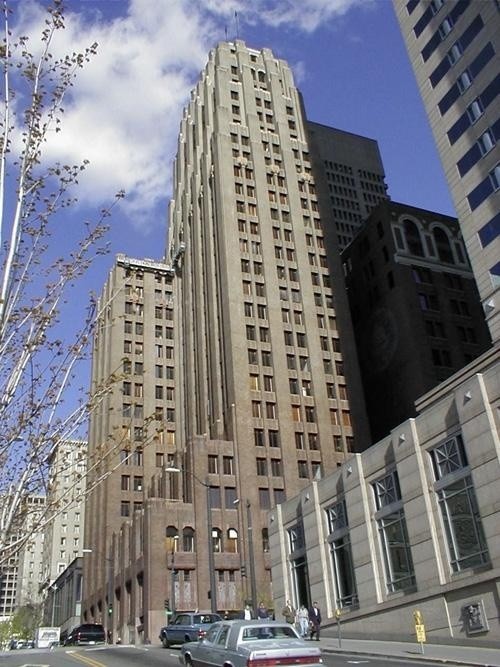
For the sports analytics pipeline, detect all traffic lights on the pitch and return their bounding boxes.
[108,602,112,614]
[164,598,168,609]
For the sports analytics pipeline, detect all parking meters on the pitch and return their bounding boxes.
[413,611,425,653]
[335,609,341,648]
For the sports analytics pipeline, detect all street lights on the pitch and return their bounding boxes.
[233,496,258,619]
[38,580,58,626]
[164,467,217,610]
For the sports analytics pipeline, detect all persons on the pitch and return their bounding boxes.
[223,599,322,641]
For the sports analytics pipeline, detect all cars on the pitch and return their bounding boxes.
[179,619,322,666]
[159,611,223,646]
[64,623,105,645]
[203,609,244,622]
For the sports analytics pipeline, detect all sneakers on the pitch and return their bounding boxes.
[310,638,320,640]
